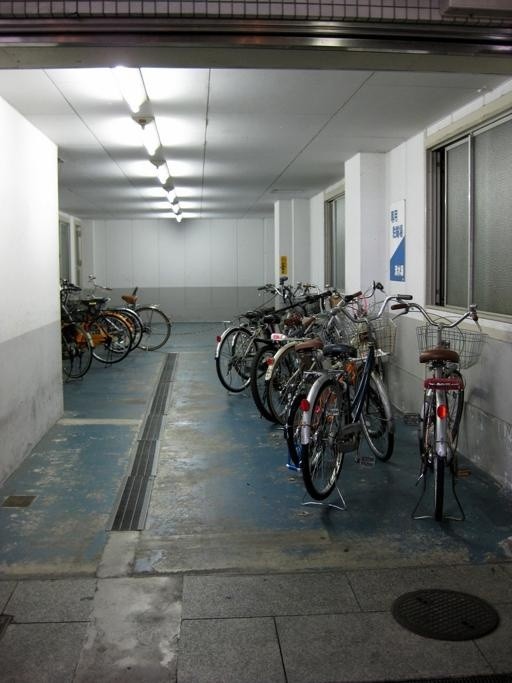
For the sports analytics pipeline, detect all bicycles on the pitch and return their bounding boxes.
[390,303,488,523]
[60,274,172,384]
